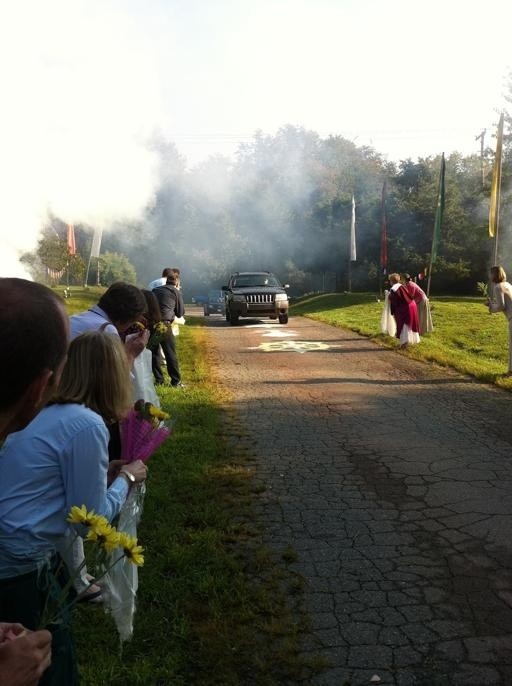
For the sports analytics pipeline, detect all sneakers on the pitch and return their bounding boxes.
[155,360,187,388]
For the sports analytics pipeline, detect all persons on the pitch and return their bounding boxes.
[1,266,186,686]
[488,264,512,375]
[381,272,433,350]
[261,276,271,285]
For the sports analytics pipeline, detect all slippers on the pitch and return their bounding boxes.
[69,578,105,604]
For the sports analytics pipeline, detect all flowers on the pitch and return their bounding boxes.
[135,320,168,349]
[38,502,146,632]
[129,399,171,437]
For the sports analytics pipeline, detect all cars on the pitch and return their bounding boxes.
[192,271,289,325]
